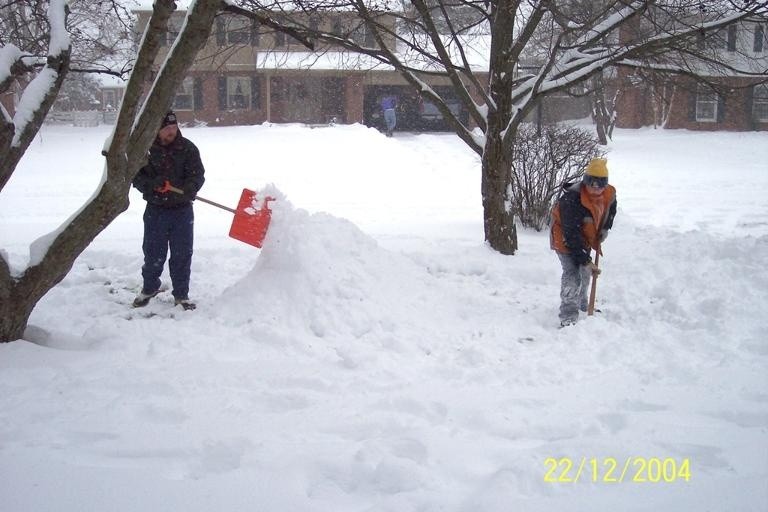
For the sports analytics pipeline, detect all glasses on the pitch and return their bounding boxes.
[582,174,608,187]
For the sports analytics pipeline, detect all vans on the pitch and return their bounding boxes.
[412,90,464,130]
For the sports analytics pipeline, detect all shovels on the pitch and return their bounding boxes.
[153,179,276,249]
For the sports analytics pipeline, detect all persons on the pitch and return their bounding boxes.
[132,110,205,309]
[550,159,617,326]
[382,93,397,137]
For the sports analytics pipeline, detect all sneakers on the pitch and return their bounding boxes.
[174,300,194,311]
[132,292,158,307]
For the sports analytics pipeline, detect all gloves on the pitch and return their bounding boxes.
[148,175,168,191]
[599,229,608,241]
[184,183,196,199]
[584,261,600,278]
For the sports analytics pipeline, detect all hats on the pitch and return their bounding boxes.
[582,158,608,187]
[160,110,177,130]
